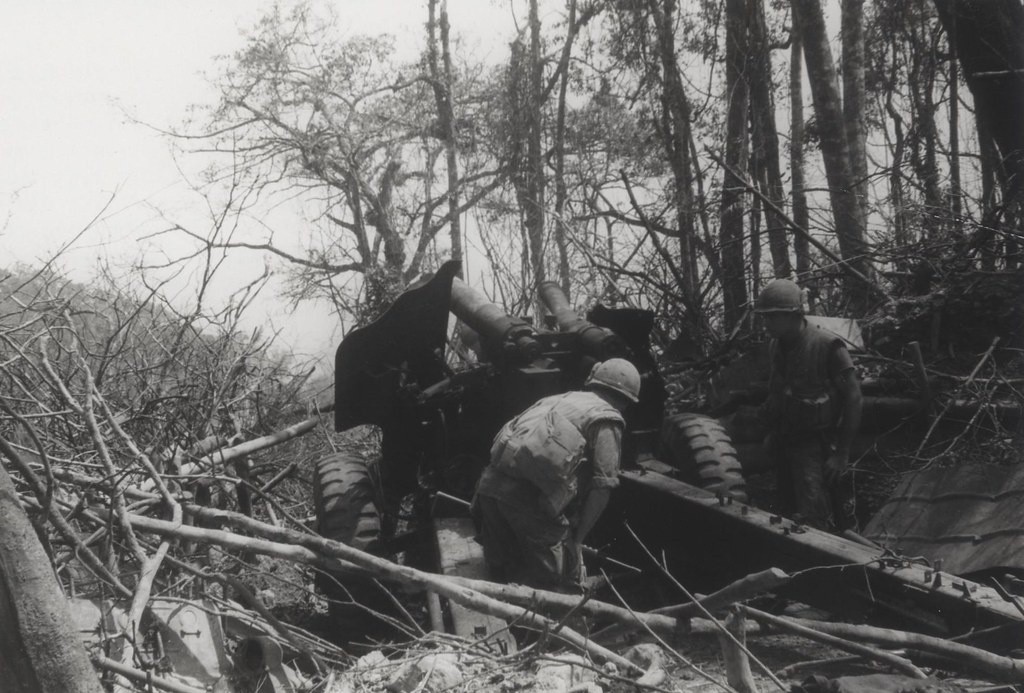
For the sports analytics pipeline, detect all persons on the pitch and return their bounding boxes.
[754,279,864,535]
[473,359,641,643]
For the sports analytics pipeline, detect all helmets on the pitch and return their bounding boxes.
[753,279,803,313]
[585,357,640,403]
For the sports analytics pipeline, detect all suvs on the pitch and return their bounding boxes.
[312,279,749,603]
